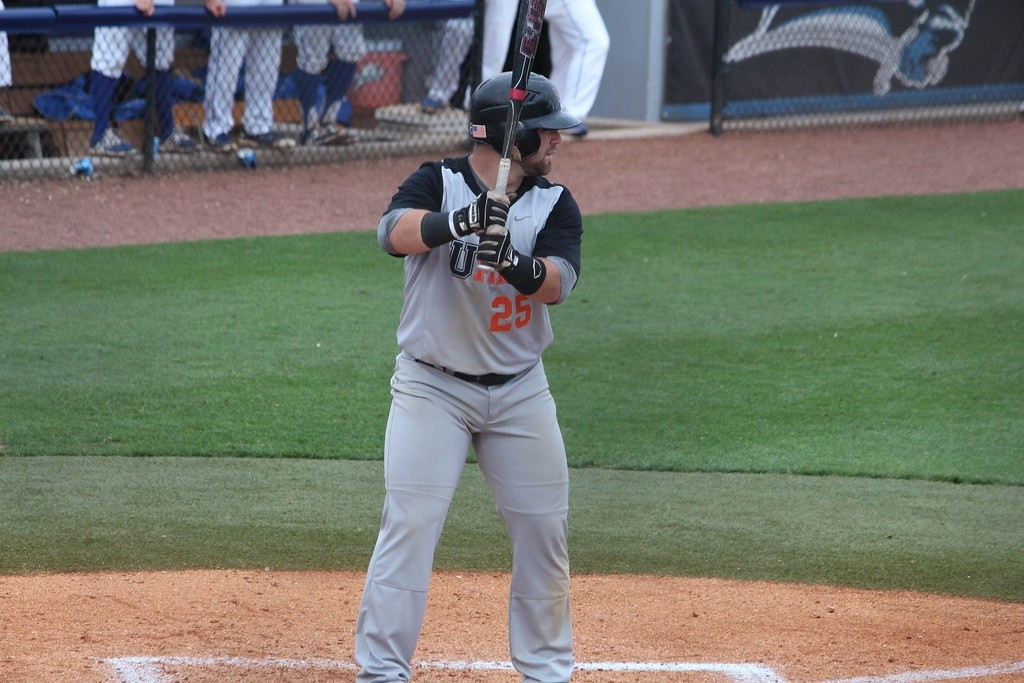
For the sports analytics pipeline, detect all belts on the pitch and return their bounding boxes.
[415,358,516,387]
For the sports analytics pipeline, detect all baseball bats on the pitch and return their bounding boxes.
[476,0,551,273]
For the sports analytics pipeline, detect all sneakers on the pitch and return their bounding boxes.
[237,127,296,150]
[198,127,239,153]
[320,124,357,146]
[88,128,137,156]
[158,124,203,154]
[557,115,589,137]
[299,121,337,146]
[421,95,445,115]
[0,109,12,123]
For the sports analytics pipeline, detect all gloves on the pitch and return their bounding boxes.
[475,224,547,296]
[420,190,510,249]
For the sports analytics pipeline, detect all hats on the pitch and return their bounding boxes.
[468,70,588,160]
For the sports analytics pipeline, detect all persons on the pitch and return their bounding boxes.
[87,0,204,156]
[356,73,583,683]
[287,0,408,147]
[199,0,297,152]
[462,0,610,136]
[421,17,475,113]
[0,1,14,121]
